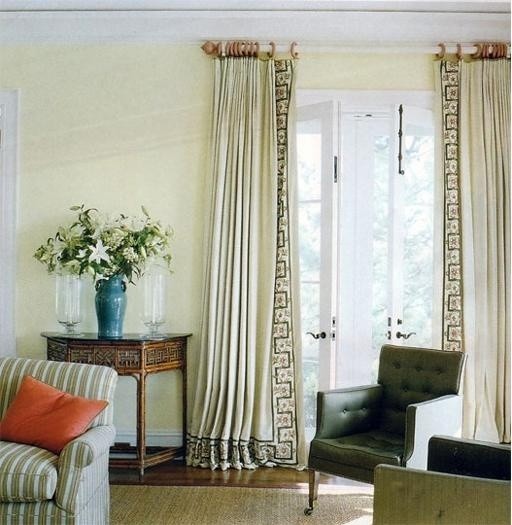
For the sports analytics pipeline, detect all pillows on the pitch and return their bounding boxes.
[0,376,110,456]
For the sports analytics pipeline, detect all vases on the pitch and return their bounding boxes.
[94,274,128,339]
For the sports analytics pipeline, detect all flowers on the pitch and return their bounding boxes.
[31,202,180,287]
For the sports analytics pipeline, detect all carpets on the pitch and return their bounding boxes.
[109,484,374,525]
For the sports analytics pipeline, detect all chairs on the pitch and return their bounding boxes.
[304,343,510,525]
[1,356,118,525]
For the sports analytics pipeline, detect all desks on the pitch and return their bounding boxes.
[41,331,193,484]
